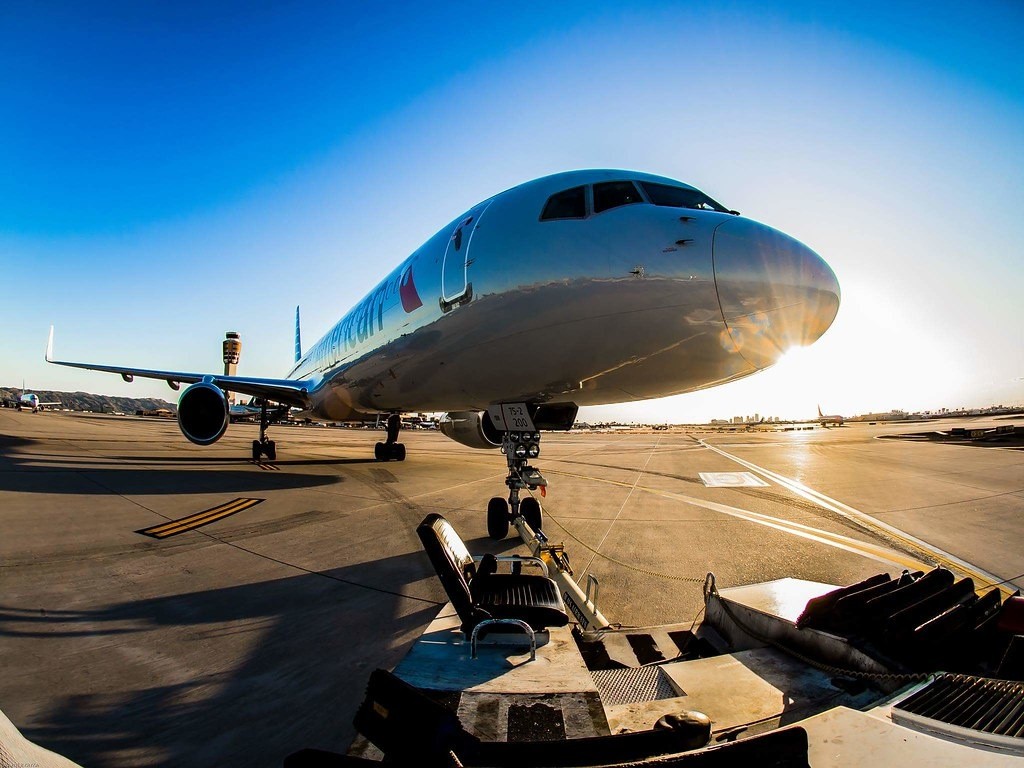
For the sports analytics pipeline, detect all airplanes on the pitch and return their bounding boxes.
[10,377,63,413]
[42,163,847,639]
[813,401,845,429]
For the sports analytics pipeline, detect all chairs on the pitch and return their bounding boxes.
[416,512,570,662]
